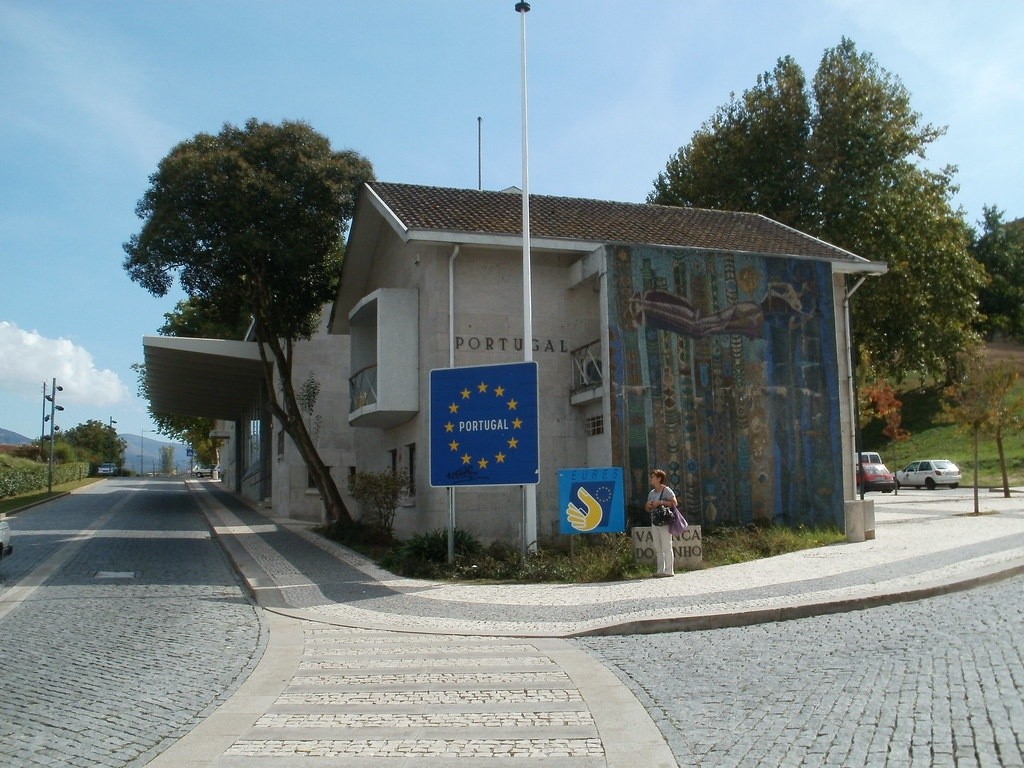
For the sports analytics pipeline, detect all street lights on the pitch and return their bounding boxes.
[107,415,118,477]
[141,427,156,476]
[475,116,483,191]
[39,381,53,457]
[50,378,65,493]
[511,1,546,560]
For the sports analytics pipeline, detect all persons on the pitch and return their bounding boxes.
[645,469,679,578]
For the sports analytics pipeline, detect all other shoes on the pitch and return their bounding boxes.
[653,573,674,577]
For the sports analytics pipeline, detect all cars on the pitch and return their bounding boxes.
[854,452,895,492]
[889,459,960,494]
[97,463,120,476]
[193,463,222,478]
[0,520,14,561]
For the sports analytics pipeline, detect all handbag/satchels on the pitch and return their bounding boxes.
[652,500,688,535]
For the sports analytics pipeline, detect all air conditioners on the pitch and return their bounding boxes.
[580,357,603,385]
